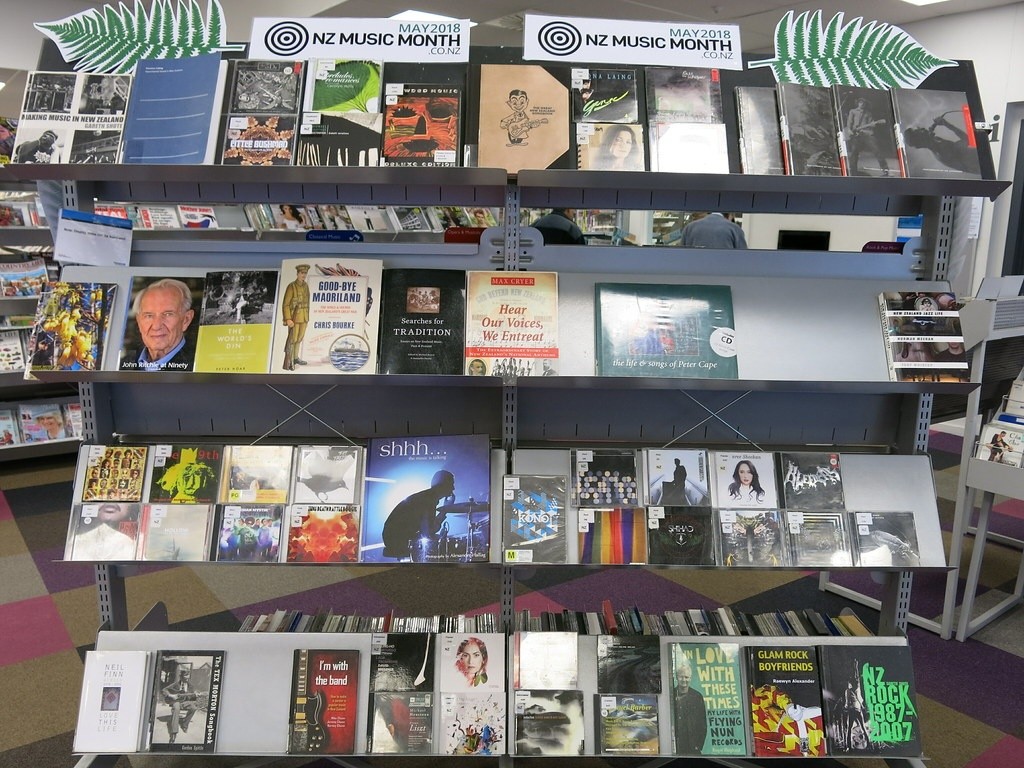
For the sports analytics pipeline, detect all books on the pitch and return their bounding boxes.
[979,413,1024,468]
[0,51,987,768]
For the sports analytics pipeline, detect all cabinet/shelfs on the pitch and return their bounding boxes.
[0,159,1024,768]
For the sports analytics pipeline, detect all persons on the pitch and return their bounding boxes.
[93,503,132,522]
[844,677,869,751]
[936,342,965,362]
[728,460,765,505]
[119,278,194,371]
[162,670,197,743]
[904,116,981,175]
[597,125,638,171]
[474,210,489,227]
[278,205,306,229]
[37,75,50,108]
[19,130,58,163]
[847,97,890,176]
[37,412,65,439]
[542,359,557,376]
[382,470,456,548]
[680,212,747,249]
[529,208,586,245]
[470,359,484,375]
[90,77,114,114]
[456,637,488,674]
[673,666,707,754]
[323,205,349,230]
[988,430,1008,461]
